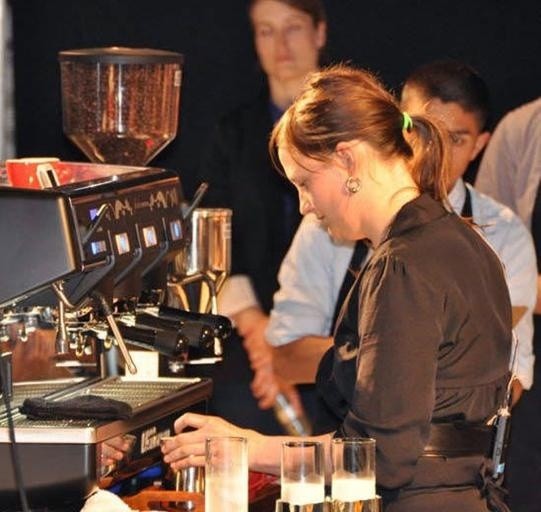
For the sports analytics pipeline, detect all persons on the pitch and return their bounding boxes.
[264,60,539,410]
[191,0,318,436]
[160,60,513,511]
[474,96,540,512]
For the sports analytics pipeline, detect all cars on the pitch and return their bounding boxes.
[280,439,326,506]
[329,438,377,501]
[202,436,252,512]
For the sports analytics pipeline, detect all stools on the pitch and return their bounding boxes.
[424,420,498,458]
